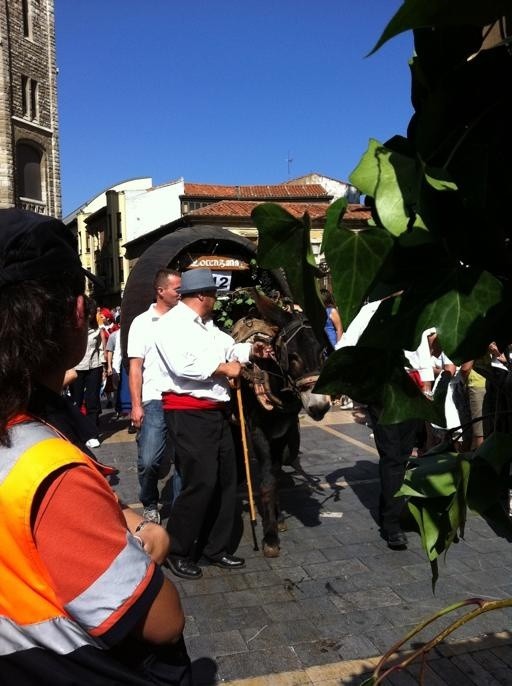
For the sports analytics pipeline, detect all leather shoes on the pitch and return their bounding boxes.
[166,556,204,581]
[381,526,408,549]
[209,551,248,570]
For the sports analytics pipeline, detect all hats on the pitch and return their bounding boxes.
[173,267,224,294]
[100,307,113,319]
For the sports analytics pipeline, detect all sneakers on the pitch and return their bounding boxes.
[84,438,101,448]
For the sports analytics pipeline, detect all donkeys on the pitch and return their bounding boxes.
[224,288,336,561]
